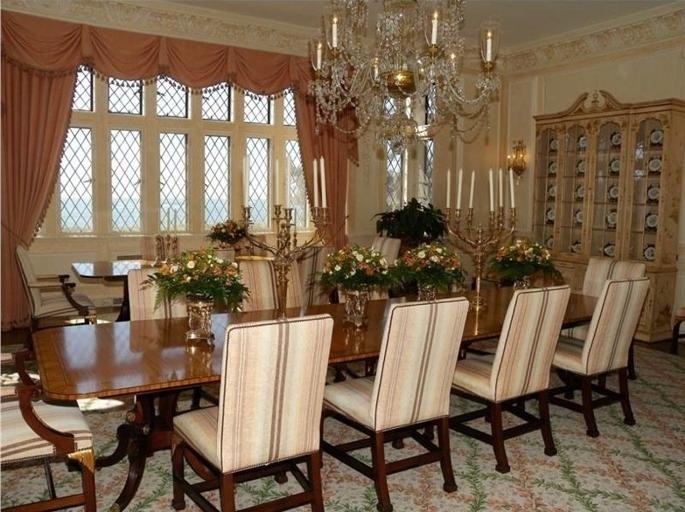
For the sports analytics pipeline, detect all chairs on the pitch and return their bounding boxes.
[1,351,97,512]
[671,308,685,353]
[127,270,188,321]
[561,258,646,380]
[421,283,571,474]
[552,277,650,437]
[170,314,335,512]
[14,245,97,332]
[320,297,469,511]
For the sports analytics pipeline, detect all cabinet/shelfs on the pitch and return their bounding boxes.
[533,91,685,343]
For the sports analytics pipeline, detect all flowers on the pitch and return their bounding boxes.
[489,236,561,280]
[303,242,401,291]
[394,242,468,283]
[205,220,246,245]
[137,248,252,313]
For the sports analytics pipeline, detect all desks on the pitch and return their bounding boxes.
[71,257,164,322]
[31,285,600,511]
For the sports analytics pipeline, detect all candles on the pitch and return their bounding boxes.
[312,158,319,208]
[455,168,463,209]
[273,157,280,205]
[468,170,476,208]
[488,168,495,212]
[157,206,161,236]
[167,207,170,234]
[319,155,327,209]
[445,168,451,208]
[498,168,504,207]
[242,156,250,208]
[174,208,177,234]
[509,167,515,208]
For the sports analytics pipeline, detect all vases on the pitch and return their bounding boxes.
[342,284,369,328]
[184,297,214,346]
[513,275,531,292]
[416,281,439,301]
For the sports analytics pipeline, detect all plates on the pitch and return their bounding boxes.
[546,129,663,261]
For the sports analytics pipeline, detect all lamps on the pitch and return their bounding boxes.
[507,140,527,186]
[305,0,501,155]
